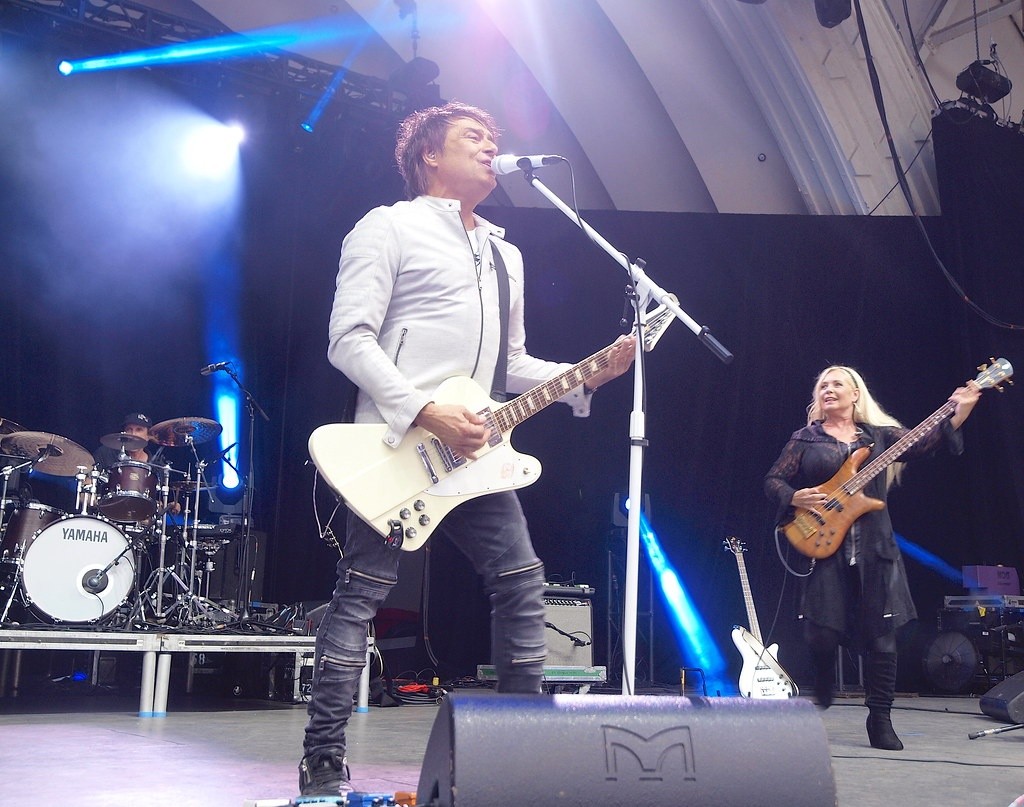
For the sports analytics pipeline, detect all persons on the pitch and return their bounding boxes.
[764,367,982,750]
[298,104,637,793]
[79,412,181,513]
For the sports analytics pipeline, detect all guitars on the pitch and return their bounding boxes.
[308,293,681,551]
[781,356,1015,559]
[720,537,800,701]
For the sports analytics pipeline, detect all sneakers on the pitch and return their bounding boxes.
[299,753,354,796]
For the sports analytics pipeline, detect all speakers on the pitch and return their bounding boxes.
[979,670,1024,725]
[175,525,267,603]
[417,690,837,807]
[540,596,592,668]
[261,601,330,636]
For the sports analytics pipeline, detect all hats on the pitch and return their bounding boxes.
[121,412,153,428]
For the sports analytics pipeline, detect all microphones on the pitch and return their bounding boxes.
[200,360,230,376]
[149,446,164,463]
[490,153,563,176]
[211,443,237,463]
[87,575,101,589]
[574,641,591,646]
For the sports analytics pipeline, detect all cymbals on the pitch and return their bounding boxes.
[147,417,223,447]
[166,479,212,491]
[0,431,96,477]
[100,433,147,451]
[1,417,28,433]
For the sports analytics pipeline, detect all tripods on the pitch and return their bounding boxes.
[130,435,307,635]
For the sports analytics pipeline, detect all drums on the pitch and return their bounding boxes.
[15,514,137,627]
[96,461,159,523]
[0,502,70,568]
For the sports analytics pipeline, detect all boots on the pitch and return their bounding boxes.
[810,650,836,709]
[865,650,903,750]
